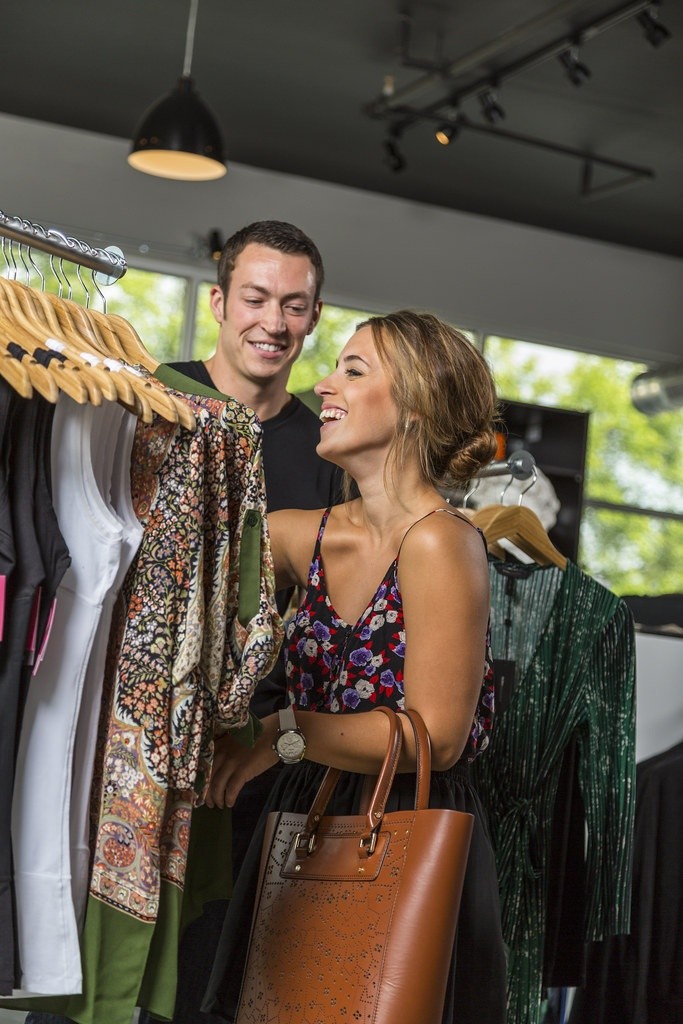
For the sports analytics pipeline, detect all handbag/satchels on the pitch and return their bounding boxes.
[234,706,475,1024]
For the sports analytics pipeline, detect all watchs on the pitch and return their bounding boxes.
[272,709,306,764]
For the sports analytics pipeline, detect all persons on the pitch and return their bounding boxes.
[196,311,503,1024]
[162,221,360,619]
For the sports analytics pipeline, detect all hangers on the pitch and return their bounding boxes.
[451,451,567,568]
[0,212,199,433]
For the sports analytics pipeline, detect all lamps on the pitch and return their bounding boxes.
[367,1,675,174]
[128,0,230,183]
